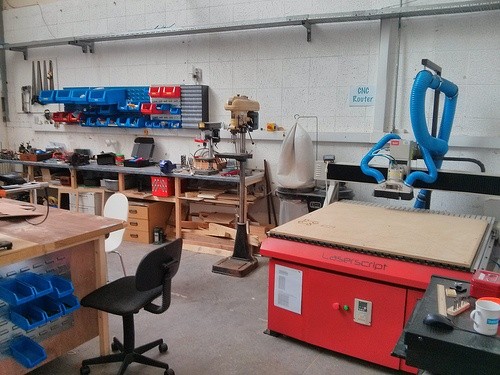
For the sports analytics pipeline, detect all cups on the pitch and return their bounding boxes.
[470,300,500,336]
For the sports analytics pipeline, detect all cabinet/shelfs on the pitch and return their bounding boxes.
[0,159,266,257]
[69,193,113,215]
[123,201,173,243]
[391,275,500,375]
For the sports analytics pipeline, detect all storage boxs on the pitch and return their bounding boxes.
[84,177,100,186]
[97,152,116,165]
[152,176,175,197]
[40,168,55,182]
[124,137,155,167]
[60,176,71,185]
[470,270,500,298]
[103,176,137,191]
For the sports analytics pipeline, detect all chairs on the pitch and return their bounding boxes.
[103,193,130,276]
[81,238,183,375]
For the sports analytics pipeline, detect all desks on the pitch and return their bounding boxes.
[0,196,129,375]
[0,182,48,205]
